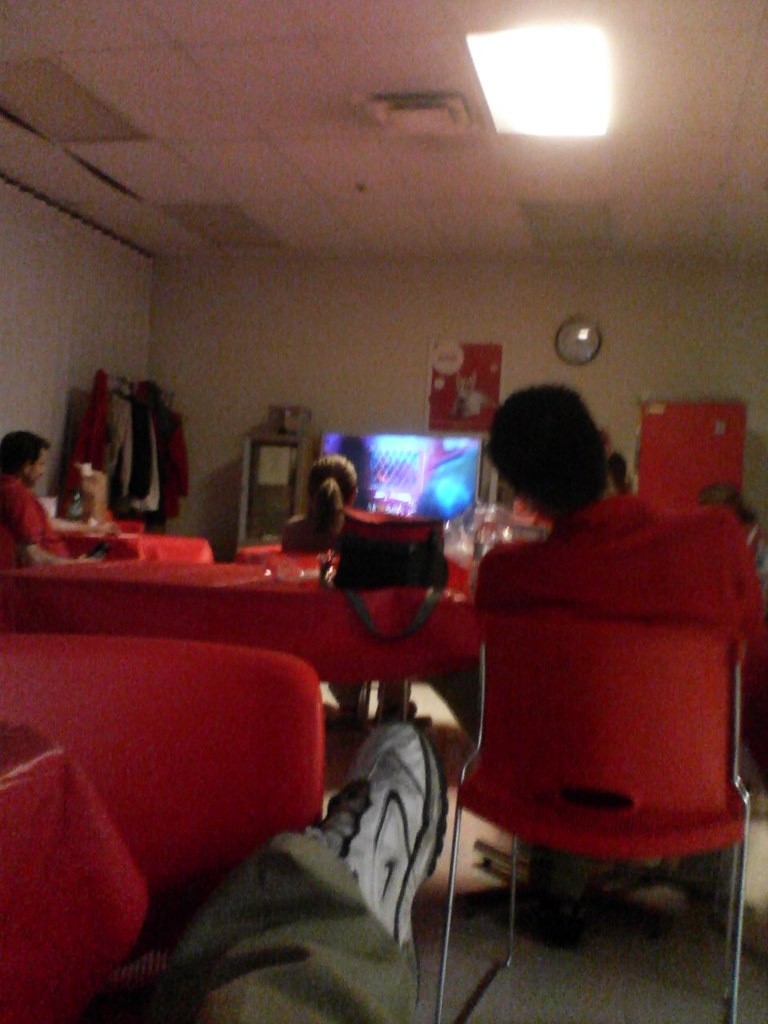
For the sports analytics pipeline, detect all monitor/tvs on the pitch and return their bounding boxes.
[320,430,485,523]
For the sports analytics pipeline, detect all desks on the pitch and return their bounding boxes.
[0,633,327,883]
[0,560,482,682]
[0,724,150,1023]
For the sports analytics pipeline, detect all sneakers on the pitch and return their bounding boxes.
[303,723,449,1009]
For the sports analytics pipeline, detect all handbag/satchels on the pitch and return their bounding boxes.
[323,509,448,644]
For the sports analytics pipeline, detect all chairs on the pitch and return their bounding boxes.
[434,600,752,1023]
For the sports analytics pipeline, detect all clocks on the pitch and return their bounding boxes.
[555,318,600,366]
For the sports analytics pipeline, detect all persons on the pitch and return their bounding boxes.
[0,430,122,570]
[281,454,417,723]
[168,719,449,1024]
[472,383,768,692]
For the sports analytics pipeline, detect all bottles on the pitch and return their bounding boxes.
[67,488,83,521]
[471,504,499,585]
